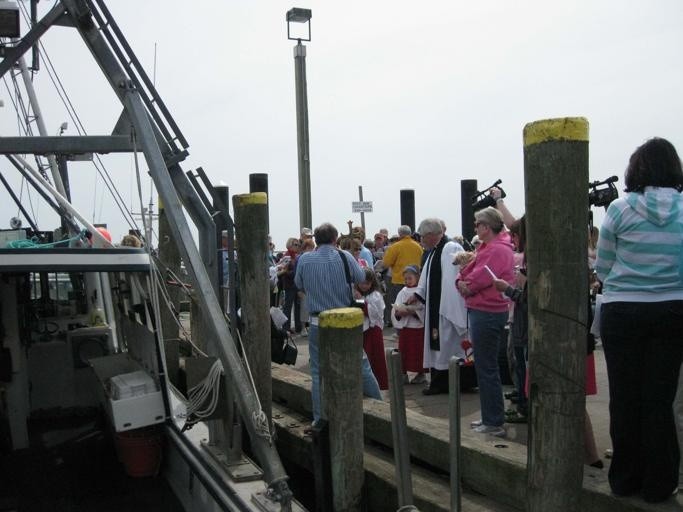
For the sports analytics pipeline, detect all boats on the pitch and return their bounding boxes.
[0,245,313,511]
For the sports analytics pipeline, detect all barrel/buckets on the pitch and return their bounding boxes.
[115,432,156,477]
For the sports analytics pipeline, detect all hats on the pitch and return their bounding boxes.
[301,228,314,236]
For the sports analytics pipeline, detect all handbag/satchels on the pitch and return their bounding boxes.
[282,342,298,366]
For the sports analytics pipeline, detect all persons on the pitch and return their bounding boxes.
[673,364,683,507]
[594,135,683,502]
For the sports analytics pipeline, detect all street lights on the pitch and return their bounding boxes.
[286,8,313,236]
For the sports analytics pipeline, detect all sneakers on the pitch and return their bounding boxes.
[421,386,449,393]
[471,417,506,437]
[402,369,431,384]
[458,384,480,394]
[503,389,528,425]
